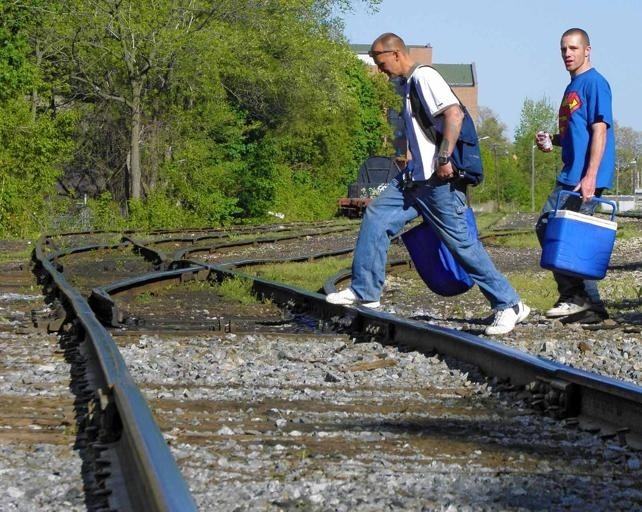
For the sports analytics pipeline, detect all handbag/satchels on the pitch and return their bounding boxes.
[400,205,481,298]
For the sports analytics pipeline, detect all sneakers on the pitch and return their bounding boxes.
[545,294,594,319]
[560,305,608,325]
[483,302,531,336]
[324,287,382,310]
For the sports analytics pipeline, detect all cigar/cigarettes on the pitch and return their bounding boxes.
[579,194,596,199]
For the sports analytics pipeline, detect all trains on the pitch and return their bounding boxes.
[336,155,401,220]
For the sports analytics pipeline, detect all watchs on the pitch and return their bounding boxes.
[436,155,449,166]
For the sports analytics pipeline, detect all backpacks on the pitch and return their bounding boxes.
[409,65,484,187]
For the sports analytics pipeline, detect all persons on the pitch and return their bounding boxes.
[536,28,617,325]
[323,32,532,335]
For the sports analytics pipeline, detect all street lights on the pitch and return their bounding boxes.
[491,142,509,210]
[479,135,489,141]
[616,159,636,211]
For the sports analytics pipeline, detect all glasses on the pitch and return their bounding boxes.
[367,50,396,58]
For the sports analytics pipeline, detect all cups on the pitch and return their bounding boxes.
[535,131,554,153]
